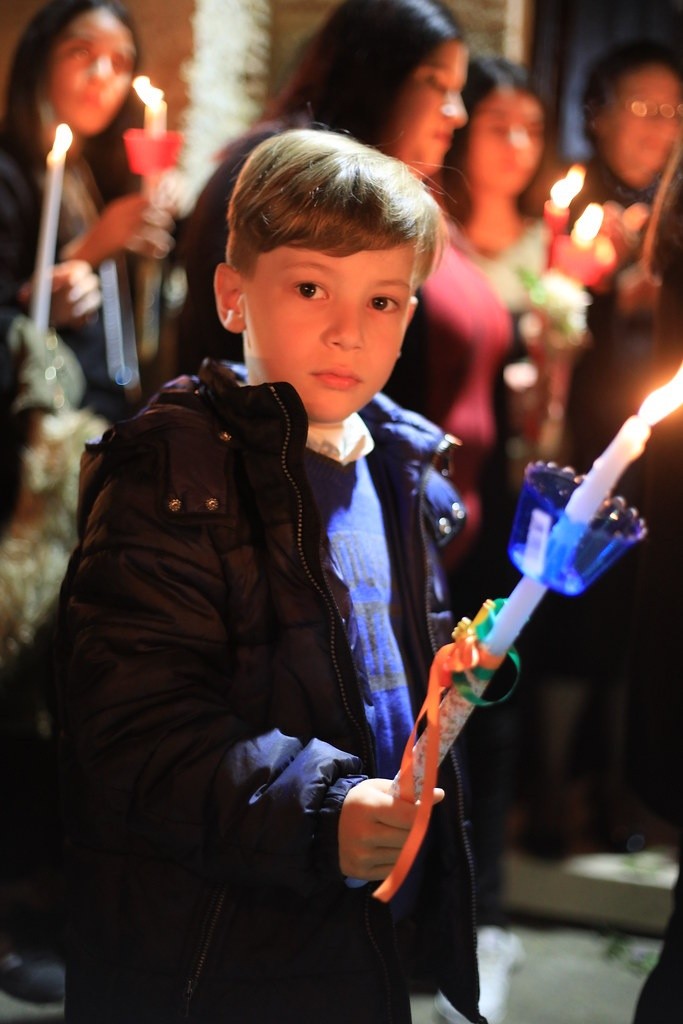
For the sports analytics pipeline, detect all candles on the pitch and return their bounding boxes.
[31,153,68,328]
[143,100,168,353]
[480,412,653,658]
[540,208,615,279]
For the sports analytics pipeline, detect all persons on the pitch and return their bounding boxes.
[1,1,682,868]
[0,123,497,1024]
